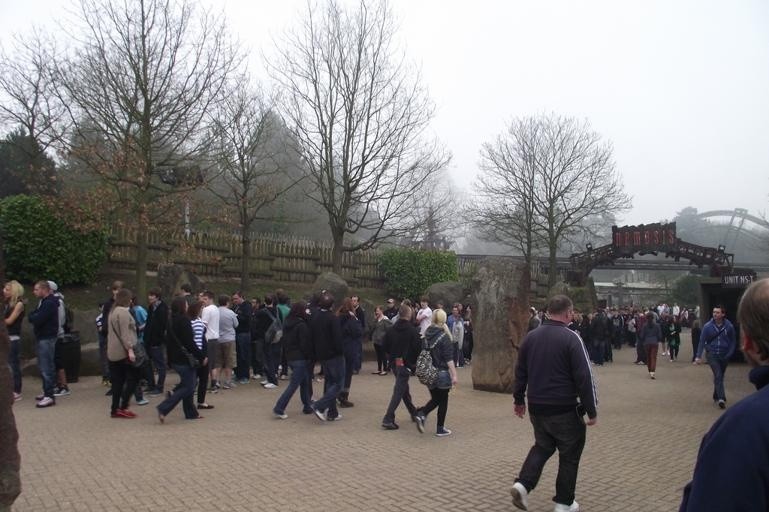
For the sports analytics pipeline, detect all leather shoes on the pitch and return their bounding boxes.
[187,415,204,418]
[372,371,387,375]
[157,406,164,423]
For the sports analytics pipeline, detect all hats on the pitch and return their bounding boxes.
[47,282,57,290]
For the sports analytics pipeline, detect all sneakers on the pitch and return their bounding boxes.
[662,350,677,361]
[554,501,579,512]
[381,421,399,431]
[102,377,112,395]
[511,483,529,512]
[143,384,175,397]
[413,406,426,433]
[13,392,23,401]
[280,375,287,380]
[253,374,277,390]
[633,361,645,365]
[197,402,214,408]
[136,399,148,404]
[37,393,53,406]
[111,406,134,418]
[207,373,248,393]
[53,384,71,397]
[650,373,656,379]
[435,429,451,437]
[272,411,287,419]
[304,401,353,422]
[719,399,725,410]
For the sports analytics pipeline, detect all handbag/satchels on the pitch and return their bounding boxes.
[60,297,73,332]
[190,353,207,377]
[126,345,149,367]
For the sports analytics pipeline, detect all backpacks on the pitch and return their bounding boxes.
[414,331,448,386]
[263,308,283,344]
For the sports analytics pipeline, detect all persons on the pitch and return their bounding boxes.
[272,291,364,422]
[528,307,549,333]
[568,303,700,379]
[1,311,23,511]
[1,279,71,408]
[510,294,597,510]
[94,281,278,423]
[696,306,736,409]
[677,277,769,511]
[370,298,474,437]
